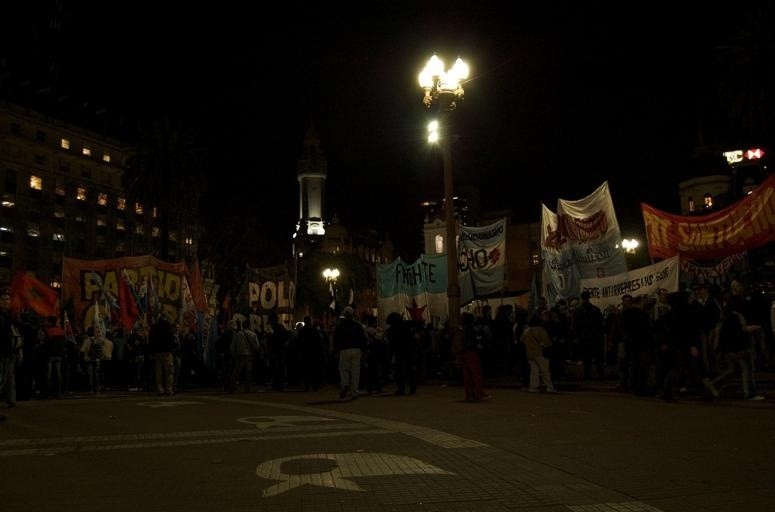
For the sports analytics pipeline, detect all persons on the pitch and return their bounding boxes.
[2,278,775,425]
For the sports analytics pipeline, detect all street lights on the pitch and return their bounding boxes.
[415,54,475,355]
[614,236,643,270]
[321,268,342,353]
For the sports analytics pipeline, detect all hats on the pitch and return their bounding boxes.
[344,307,353,314]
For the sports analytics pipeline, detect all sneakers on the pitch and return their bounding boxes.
[8,402,16,408]
[158,391,166,395]
[546,385,558,392]
[529,387,540,393]
[0,416,7,421]
[339,384,349,399]
[240,386,246,392]
[352,390,359,397]
[168,391,176,396]
[250,386,257,392]
[707,382,719,395]
[747,394,765,400]
[481,394,489,400]
